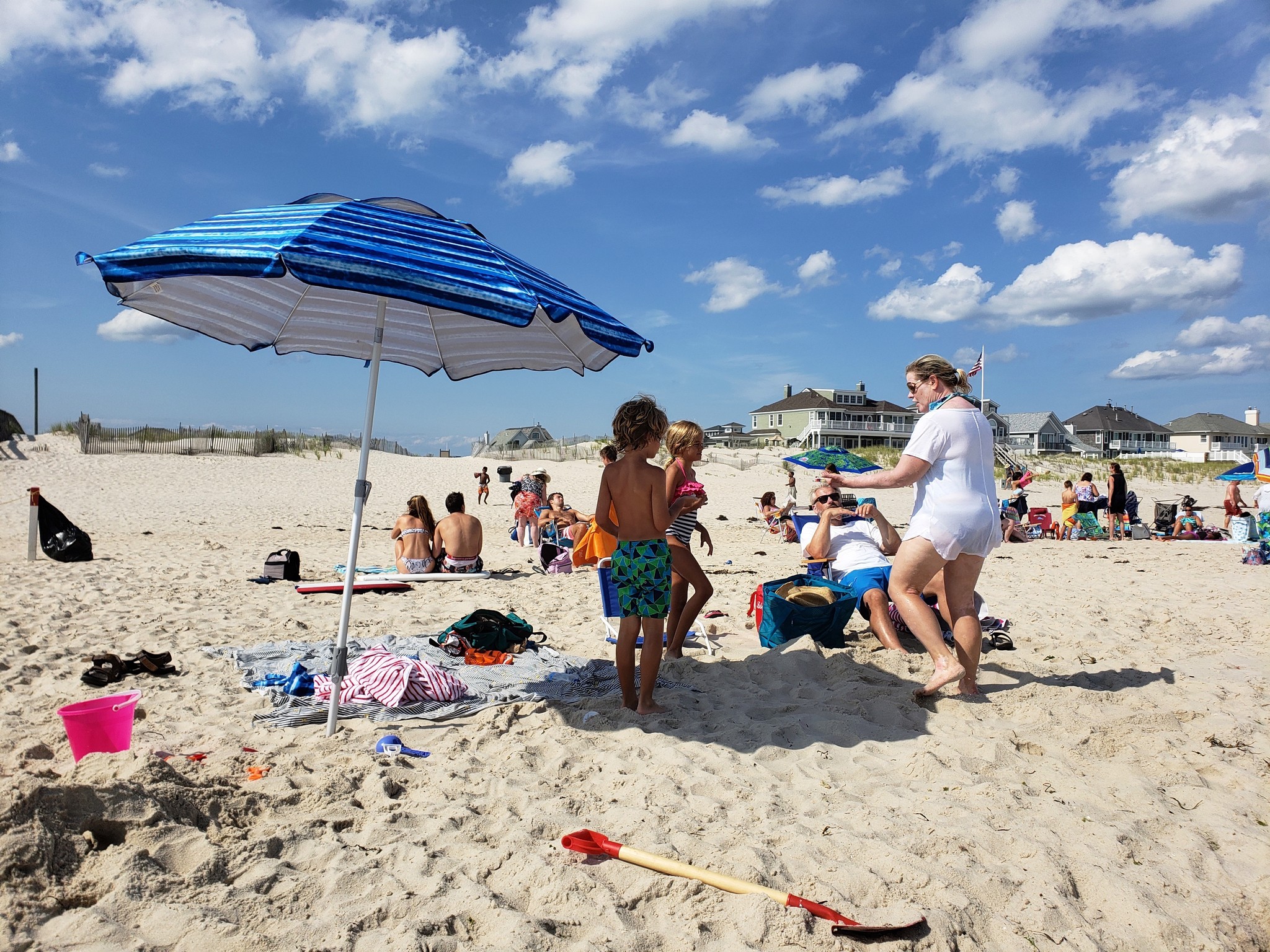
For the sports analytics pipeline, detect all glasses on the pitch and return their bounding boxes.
[770,497,776,500]
[1184,509,1192,512]
[906,376,930,393]
[407,501,411,506]
[813,492,840,505]
[1110,466,1114,469]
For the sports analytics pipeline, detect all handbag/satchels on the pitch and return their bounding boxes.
[508,523,533,546]
[1196,528,1223,540]
[437,609,547,654]
[263,549,300,580]
[746,584,763,633]
[1240,545,1268,565]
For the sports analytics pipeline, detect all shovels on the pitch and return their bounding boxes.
[563,827,926,932]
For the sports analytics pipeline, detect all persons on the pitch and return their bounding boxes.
[1223,480,1247,529]
[824,463,841,475]
[785,471,798,506]
[1152,505,1222,542]
[1252,483,1270,563]
[431,491,484,573]
[590,393,713,713]
[760,491,796,531]
[391,496,436,573]
[799,480,953,656]
[1058,463,1128,540]
[513,467,551,548]
[537,492,596,569]
[474,467,490,505]
[818,353,1003,699]
[997,464,1039,544]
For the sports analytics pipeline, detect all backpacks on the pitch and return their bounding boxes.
[538,542,573,573]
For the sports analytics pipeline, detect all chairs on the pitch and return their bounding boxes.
[1174,511,1206,536]
[789,508,868,583]
[1000,505,1042,539]
[751,496,788,544]
[1105,507,1133,538]
[597,555,712,657]
[1076,510,1119,540]
[533,502,592,547]
[1027,507,1058,539]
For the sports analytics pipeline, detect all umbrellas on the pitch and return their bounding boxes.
[780,446,884,475]
[75,192,653,735]
[1214,445,1270,484]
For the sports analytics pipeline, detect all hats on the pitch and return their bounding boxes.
[1004,464,1010,468]
[774,581,836,607]
[530,467,551,483]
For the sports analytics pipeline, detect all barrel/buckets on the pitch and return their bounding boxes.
[57,689,142,764]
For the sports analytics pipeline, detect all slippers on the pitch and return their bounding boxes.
[532,565,547,575]
[703,610,729,618]
[988,630,1014,650]
[492,568,519,574]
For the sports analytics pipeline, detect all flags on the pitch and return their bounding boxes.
[967,351,982,378]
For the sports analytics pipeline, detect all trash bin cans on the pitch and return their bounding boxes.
[497,465,513,482]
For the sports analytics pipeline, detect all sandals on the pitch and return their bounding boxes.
[121,650,175,676]
[80,654,124,687]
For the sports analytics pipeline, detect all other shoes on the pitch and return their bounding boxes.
[758,574,858,650]
